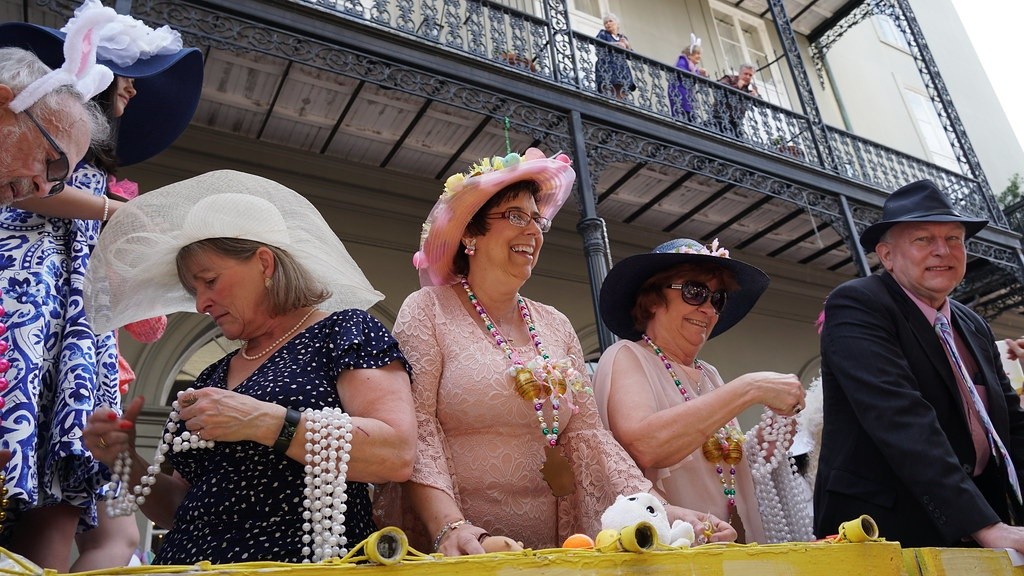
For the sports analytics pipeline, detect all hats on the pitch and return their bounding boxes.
[0,0,204,170]
[858,181,990,253]
[598,238,771,343]
[82,172,385,336]
[412,148,577,288]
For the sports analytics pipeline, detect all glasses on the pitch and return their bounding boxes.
[485,210,552,234]
[650,279,727,316]
[24,109,69,198]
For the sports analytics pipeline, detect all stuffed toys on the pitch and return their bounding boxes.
[600,492,695,549]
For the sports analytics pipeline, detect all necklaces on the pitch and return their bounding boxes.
[241,306,319,359]
[460,274,568,448]
[302,407,353,563]
[743,404,815,545]
[642,334,744,544]
[106,388,215,519]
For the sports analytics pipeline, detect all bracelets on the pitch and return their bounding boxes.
[271,408,301,454]
[433,520,472,552]
[660,500,670,506]
[102,195,109,221]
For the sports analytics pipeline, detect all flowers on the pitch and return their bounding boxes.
[412,138,573,271]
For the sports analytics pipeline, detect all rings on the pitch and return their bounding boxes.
[97,437,108,449]
[793,405,801,414]
[197,431,201,439]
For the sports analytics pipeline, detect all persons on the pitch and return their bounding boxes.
[595,13,637,101]
[667,32,709,122]
[372,147,737,557]
[712,64,763,139]
[0,0,203,573]
[812,180,1024,554]
[82,169,418,566]
[591,239,806,544]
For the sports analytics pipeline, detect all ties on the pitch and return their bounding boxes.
[933,310,1023,506]
